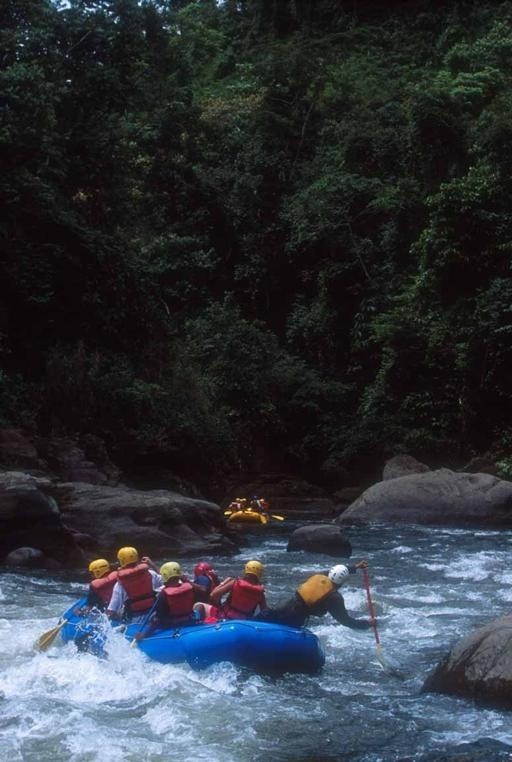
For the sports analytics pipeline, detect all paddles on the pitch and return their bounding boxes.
[270,514,284,522]
[34,597,89,652]
[359,562,386,658]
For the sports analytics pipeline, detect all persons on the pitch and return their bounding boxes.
[192,561,266,622]
[250,560,376,629]
[181,562,222,605]
[103,547,163,619]
[228,494,269,516]
[135,562,194,642]
[75,558,118,615]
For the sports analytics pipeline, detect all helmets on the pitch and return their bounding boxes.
[195,562,216,582]
[327,564,351,586]
[88,558,111,579]
[116,546,138,568]
[243,560,264,581]
[160,561,183,585]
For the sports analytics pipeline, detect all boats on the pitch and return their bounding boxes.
[230,510,272,524]
[56,593,328,674]
